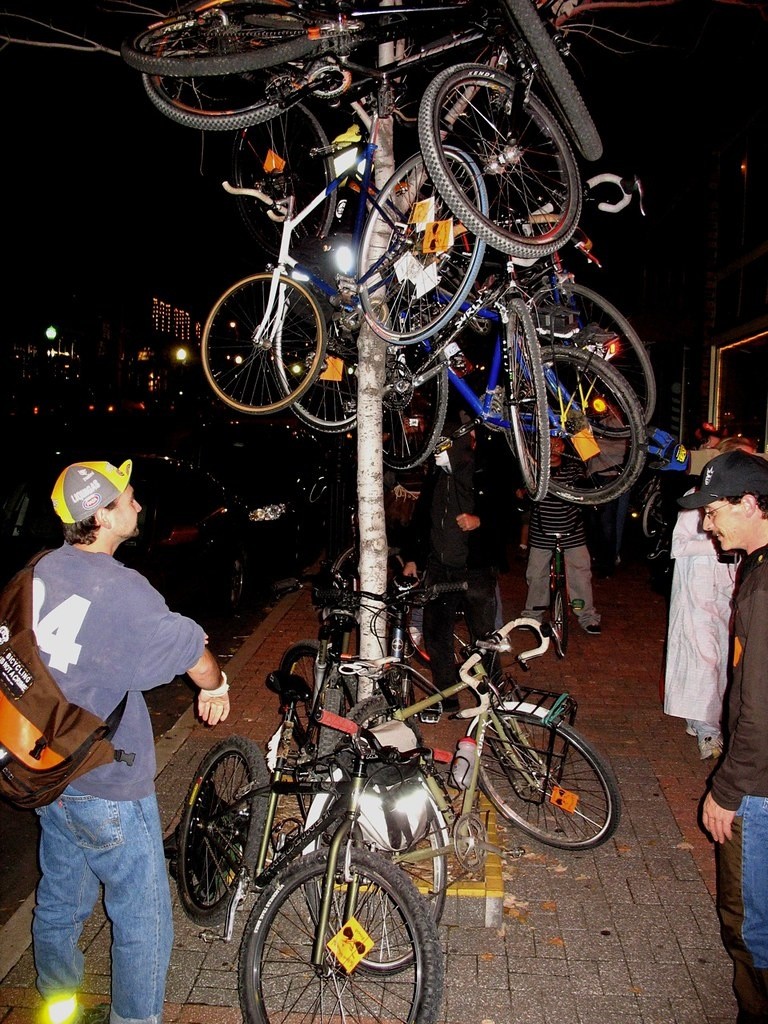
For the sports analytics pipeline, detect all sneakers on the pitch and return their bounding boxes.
[585,624,601,634]
[699,735,723,759]
[685,725,698,737]
[499,637,511,653]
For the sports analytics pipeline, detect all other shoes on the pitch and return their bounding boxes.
[419,697,460,711]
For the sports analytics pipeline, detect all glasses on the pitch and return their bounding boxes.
[704,500,740,517]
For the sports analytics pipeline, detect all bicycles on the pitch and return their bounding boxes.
[120,1,655,1024]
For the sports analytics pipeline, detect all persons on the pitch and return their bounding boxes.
[517,435,602,634]
[403,418,502,711]
[634,420,768,1023]
[32,458,230,1024]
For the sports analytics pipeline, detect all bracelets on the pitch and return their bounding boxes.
[201,671,230,698]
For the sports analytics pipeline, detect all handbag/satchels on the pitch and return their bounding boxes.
[1,547,136,807]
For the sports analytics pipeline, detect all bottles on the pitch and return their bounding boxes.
[451,736,477,789]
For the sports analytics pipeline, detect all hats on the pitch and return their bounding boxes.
[678,448,768,508]
[51,459,132,524]
